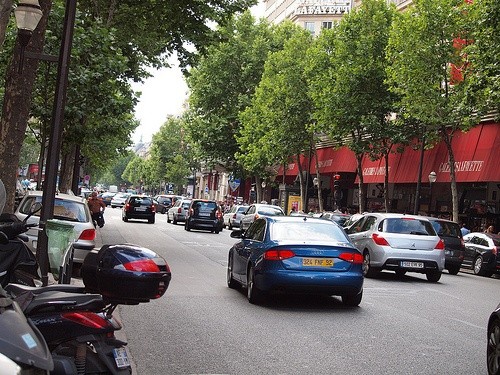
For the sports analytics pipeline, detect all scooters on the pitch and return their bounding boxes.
[0,202,172,375]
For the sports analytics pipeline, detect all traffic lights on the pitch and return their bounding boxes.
[334,175,341,189]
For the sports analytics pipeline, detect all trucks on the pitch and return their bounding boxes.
[27,163,45,184]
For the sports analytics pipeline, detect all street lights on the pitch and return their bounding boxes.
[428,171,436,217]
[313,178,318,212]
[262,182,266,200]
[12,0,75,287]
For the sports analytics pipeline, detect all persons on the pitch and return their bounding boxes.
[92,183,161,197]
[16,175,38,190]
[481,225,494,234]
[460,223,470,237]
[87,192,105,230]
[213,196,238,211]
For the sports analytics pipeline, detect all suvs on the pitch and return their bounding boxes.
[167,199,222,233]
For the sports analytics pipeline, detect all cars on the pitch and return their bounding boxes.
[9,188,103,277]
[461,232,500,276]
[223,204,286,233]
[78,186,187,224]
[24,179,45,191]
[227,216,368,307]
[486,301,500,375]
[341,212,465,276]
[289,211,352,225]
